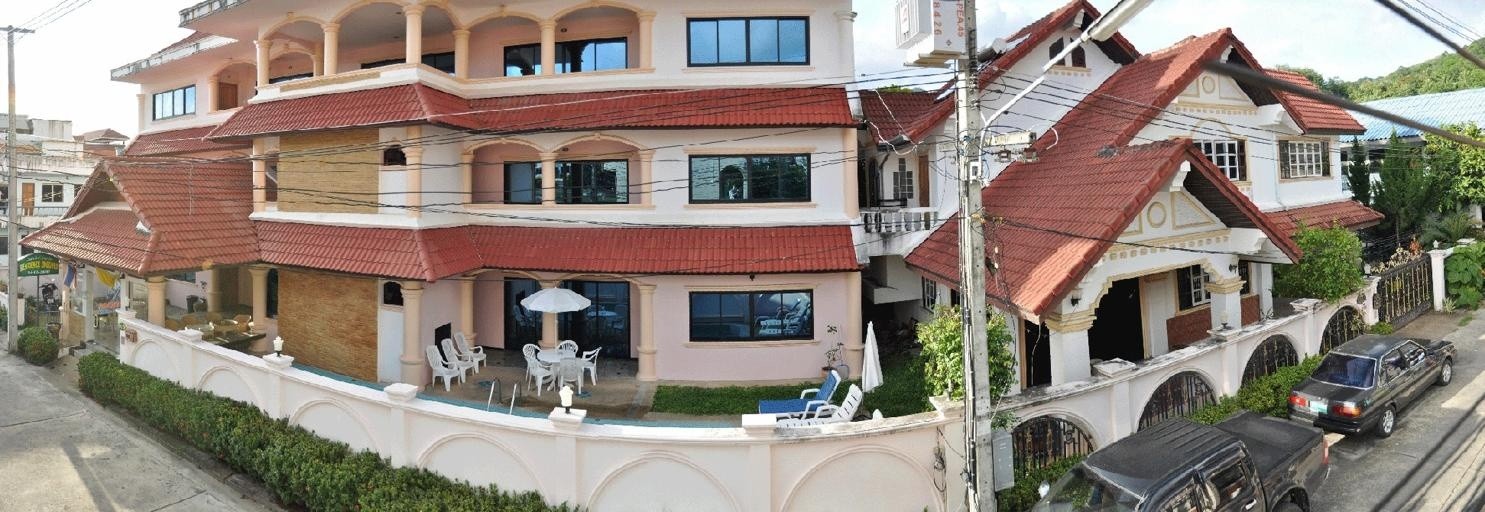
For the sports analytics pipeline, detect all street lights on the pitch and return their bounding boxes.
[948,0,1155,511]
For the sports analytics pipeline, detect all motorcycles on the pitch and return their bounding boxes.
[38,281,56,311]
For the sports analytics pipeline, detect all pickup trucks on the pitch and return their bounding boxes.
[1021,413,1328,512]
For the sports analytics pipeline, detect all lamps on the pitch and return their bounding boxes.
[1433,239,1440,250]
[1363,263,1372,279]
[558,386,574,413]
[272,334,284,357]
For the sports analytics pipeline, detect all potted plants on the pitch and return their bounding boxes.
[821,322,844,372]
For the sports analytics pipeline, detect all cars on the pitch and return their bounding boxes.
[1287,333,1457,440]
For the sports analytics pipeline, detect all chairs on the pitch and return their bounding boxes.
[522,340,602,397]
[426,344,460,392]
[453,331,487,375]
[778,383,863,429]
[440,337,475,383]
[165,310,268,350]
[758,369,841,419]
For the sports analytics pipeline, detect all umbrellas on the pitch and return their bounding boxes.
[520,286,591,354]
[861,320,884,414]
